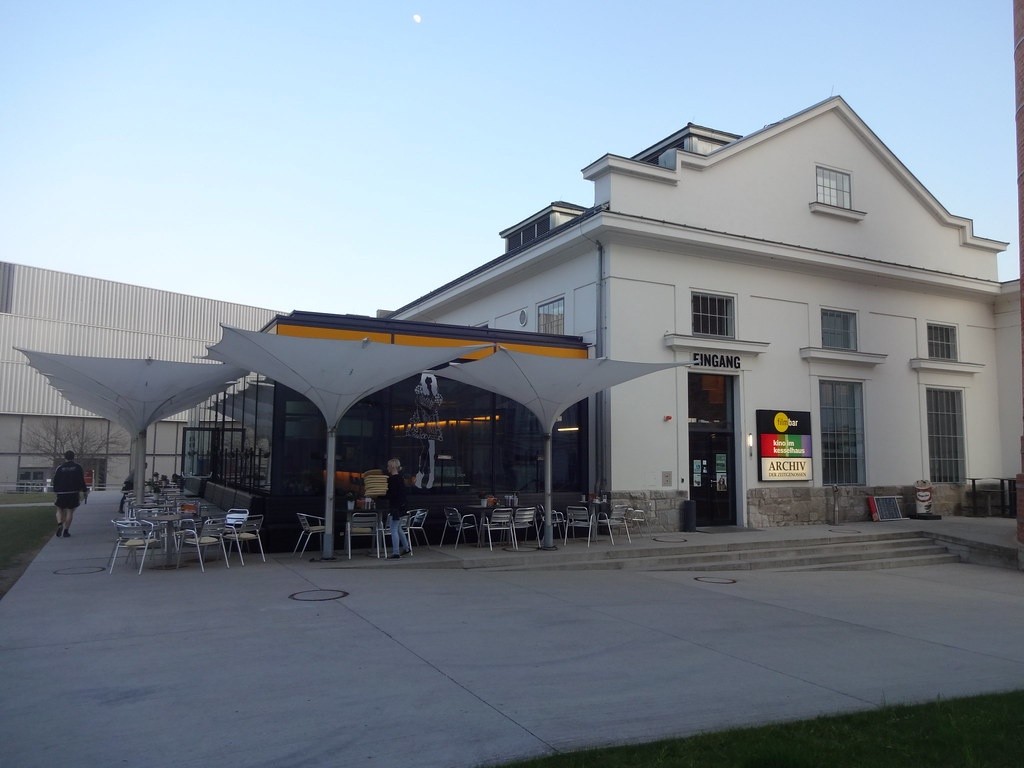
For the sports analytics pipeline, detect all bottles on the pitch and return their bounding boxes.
[513,493,518,506]
[495,498,501,508]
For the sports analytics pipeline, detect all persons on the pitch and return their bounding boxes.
[52,451,88,537]
[118,463,147,514]
[385,458,413,561]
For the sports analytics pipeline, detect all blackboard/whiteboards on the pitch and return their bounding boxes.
[873,496,902,521]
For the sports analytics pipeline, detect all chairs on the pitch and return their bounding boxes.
[291,501,652,560]
[106,480,265,575]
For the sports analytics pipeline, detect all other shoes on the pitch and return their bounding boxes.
[387,554,400,560]
[118,508,125,513]
[399,548,411,556]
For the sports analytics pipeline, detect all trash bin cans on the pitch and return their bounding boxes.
[684,500,696,533]
[914,480,933,515]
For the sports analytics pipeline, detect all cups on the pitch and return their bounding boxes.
[505,495,512,507]
[348,500,355,510]
[603,495,608,502]
[580,494,586,502]
[593,499,600,503]
[481,499,488,507]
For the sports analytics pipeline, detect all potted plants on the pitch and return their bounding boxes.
[342,487,361,510]
[145,471,183,501]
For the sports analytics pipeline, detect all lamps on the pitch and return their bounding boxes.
[748,432,752,457]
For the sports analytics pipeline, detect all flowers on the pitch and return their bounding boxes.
[478,490,488,498]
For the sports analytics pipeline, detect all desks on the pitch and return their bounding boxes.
[334,506,391,556]
[143,515,188,571]
[577,500,608,543]
[464,504,534,550]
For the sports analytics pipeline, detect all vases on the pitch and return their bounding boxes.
[480,499,487,507]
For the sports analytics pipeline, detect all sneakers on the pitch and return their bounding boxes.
[63,529,71,538]
[56,524,63,537]
